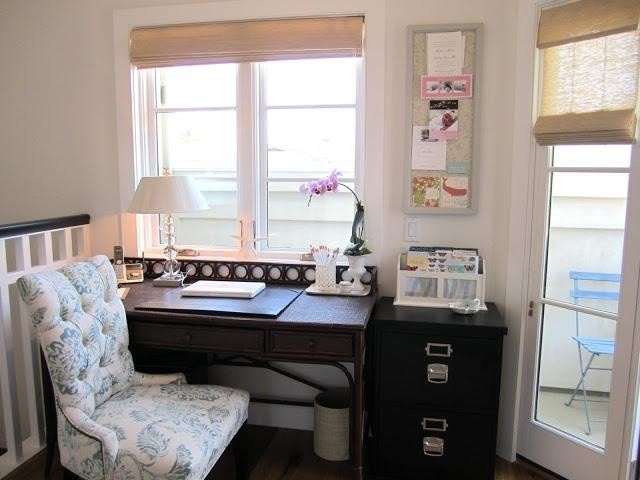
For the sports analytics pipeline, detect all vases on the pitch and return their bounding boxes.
[343,245,368,293]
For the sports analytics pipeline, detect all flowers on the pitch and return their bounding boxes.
[301,172,368,249]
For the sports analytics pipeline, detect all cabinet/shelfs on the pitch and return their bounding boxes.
[364,297,507,480]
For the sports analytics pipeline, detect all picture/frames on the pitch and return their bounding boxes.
[402,23,486,214]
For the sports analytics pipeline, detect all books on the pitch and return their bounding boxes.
[406,250,478,276]
[118,288,130,300]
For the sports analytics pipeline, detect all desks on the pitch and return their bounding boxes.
[118,272,376,480]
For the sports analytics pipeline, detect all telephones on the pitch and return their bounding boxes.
[112,245,145,284]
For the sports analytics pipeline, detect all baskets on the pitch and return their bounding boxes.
[316,264,336,292]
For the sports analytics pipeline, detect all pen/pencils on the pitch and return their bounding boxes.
[310,244,340,265]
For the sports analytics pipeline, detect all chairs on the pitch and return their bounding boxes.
[7,256,251,479]
[564,270,622,438]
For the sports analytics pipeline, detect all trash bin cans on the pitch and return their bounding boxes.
[312,389,351,462]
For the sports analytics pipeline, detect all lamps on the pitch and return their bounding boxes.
[125,168,208,288]
